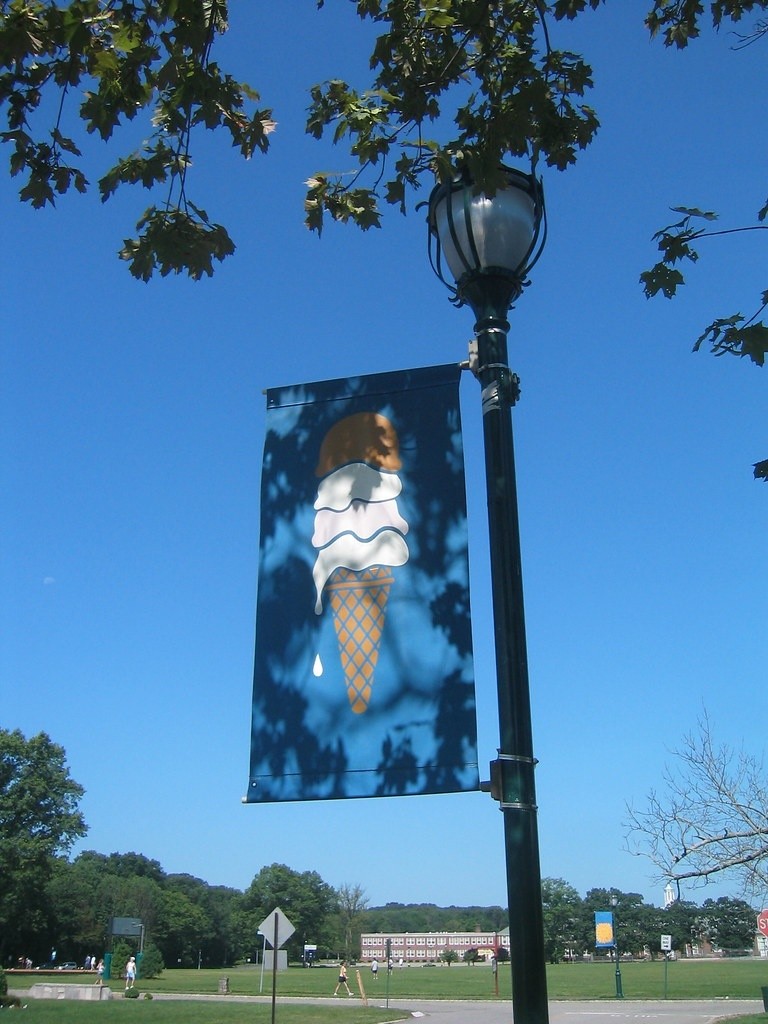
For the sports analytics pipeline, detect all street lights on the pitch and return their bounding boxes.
[416,151,550,1024]
[610,894,624,999]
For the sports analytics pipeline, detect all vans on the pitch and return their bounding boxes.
[55,961,78,971]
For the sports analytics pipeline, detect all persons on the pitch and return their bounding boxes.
[369,957,379,980]
[95,959,104,985]
[387,958,394,976]
[90,955,96,971]
[333,961,355,997]
[124,957,136,990]
[84,954,91,971]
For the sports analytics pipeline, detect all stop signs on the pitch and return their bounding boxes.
[756,910,768,938]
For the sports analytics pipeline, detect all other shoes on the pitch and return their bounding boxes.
[126,987,129,989]
[373,977,378,979]
[130,986,134,988]
[349,993,354,996]
[334,993,337,995]
